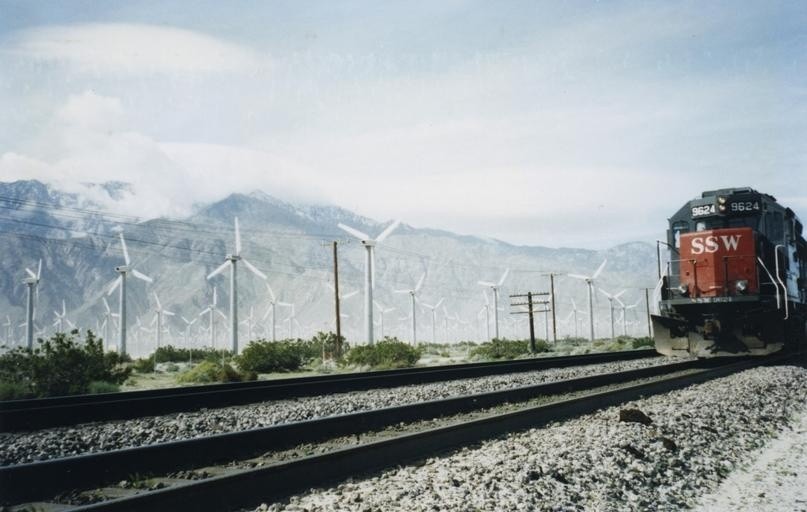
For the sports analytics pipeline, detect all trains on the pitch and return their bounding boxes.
[643,185,806,363]
